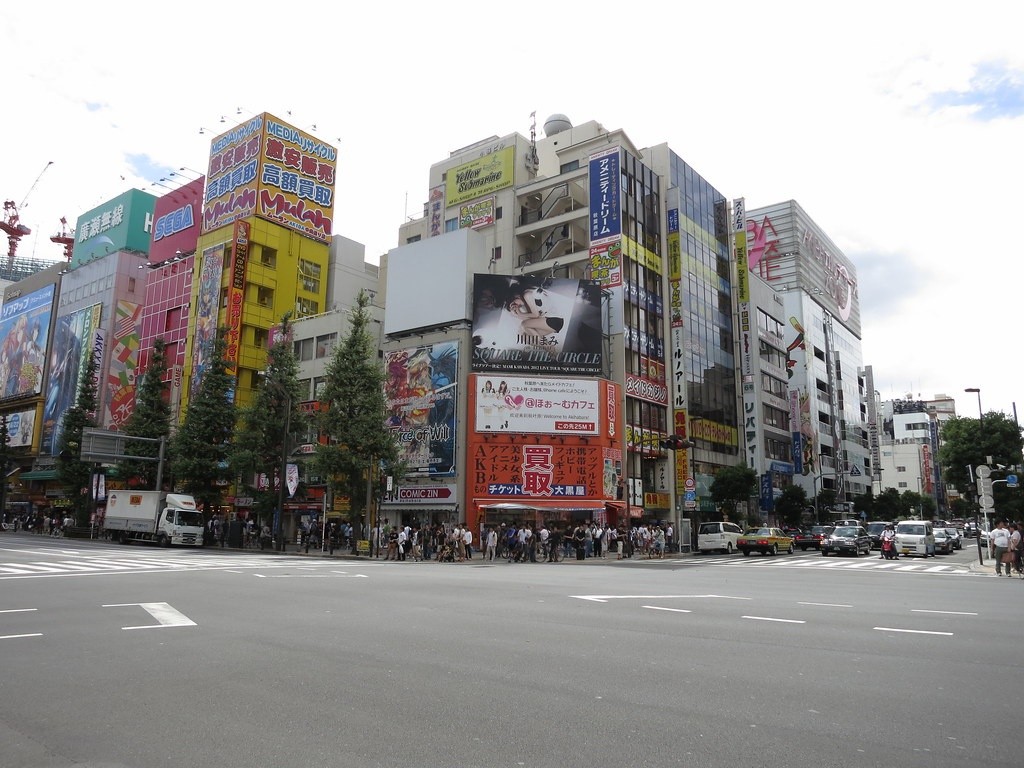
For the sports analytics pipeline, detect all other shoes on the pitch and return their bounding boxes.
[998,573,1001,576]
[895,557,899,560]
[878,556,884,559]
[1008,574,1011,577]
[601,556,608,560]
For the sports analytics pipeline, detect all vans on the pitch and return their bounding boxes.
[697,521,745,554]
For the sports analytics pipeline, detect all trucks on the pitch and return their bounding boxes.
[102,489,204,548]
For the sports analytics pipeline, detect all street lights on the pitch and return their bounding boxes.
[819,453,827,491]
[878,468,884,493]
[257,371,292,551]
[963,388,987,461]
[917,477,923,493]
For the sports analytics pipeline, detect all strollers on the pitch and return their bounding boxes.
[407,542,423,562]
[501,539,514,559]
[439,542,456,563]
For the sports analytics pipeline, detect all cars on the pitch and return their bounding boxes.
[736,527,794,556]
[933,518,965,530]
[946,528,962,550]
[833,519,897,549]
[821,526,872,557]
[933,528,954,555]
[895,520,936,558]
[796,525,834,551]
[784,528,801,537]
[980,523,993,548]
[963,516,986,538]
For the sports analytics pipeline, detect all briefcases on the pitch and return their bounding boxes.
[1001,549,1015,563]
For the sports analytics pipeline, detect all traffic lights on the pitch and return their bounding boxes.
[660,439,694,450]
[380,477,385,491]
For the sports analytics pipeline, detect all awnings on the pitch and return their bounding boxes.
[380,502,459,512]
[605,502,644,518]
[473,499,606,516]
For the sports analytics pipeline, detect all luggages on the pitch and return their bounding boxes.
[576,540,586,560]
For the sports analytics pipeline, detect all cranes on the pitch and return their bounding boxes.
[0,161,55,273]
[49,217,75,263]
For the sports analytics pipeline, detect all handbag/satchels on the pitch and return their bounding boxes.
[398,543,405,554]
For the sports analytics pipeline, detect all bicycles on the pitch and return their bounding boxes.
[649,539,666,559]
[1008,553,1024,580]
[52,526,64,539]
[535,539,566,563]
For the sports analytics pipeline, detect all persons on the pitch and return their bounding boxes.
[12,511,74,535]
[297,519,665,563]
[504,284,564,356]
[211,516,270,549]
[496,381,513,429]
[966,521,972,539]
[1009,523,1021,574]
[482,381,495,429]
[666,523,673,553]
[990,517,1012,577]
[879,524,899,560]
[1016,521,1024,579]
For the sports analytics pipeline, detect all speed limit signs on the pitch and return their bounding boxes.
[685,478,694,487]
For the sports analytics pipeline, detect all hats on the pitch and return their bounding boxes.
[619,523,626,527]
